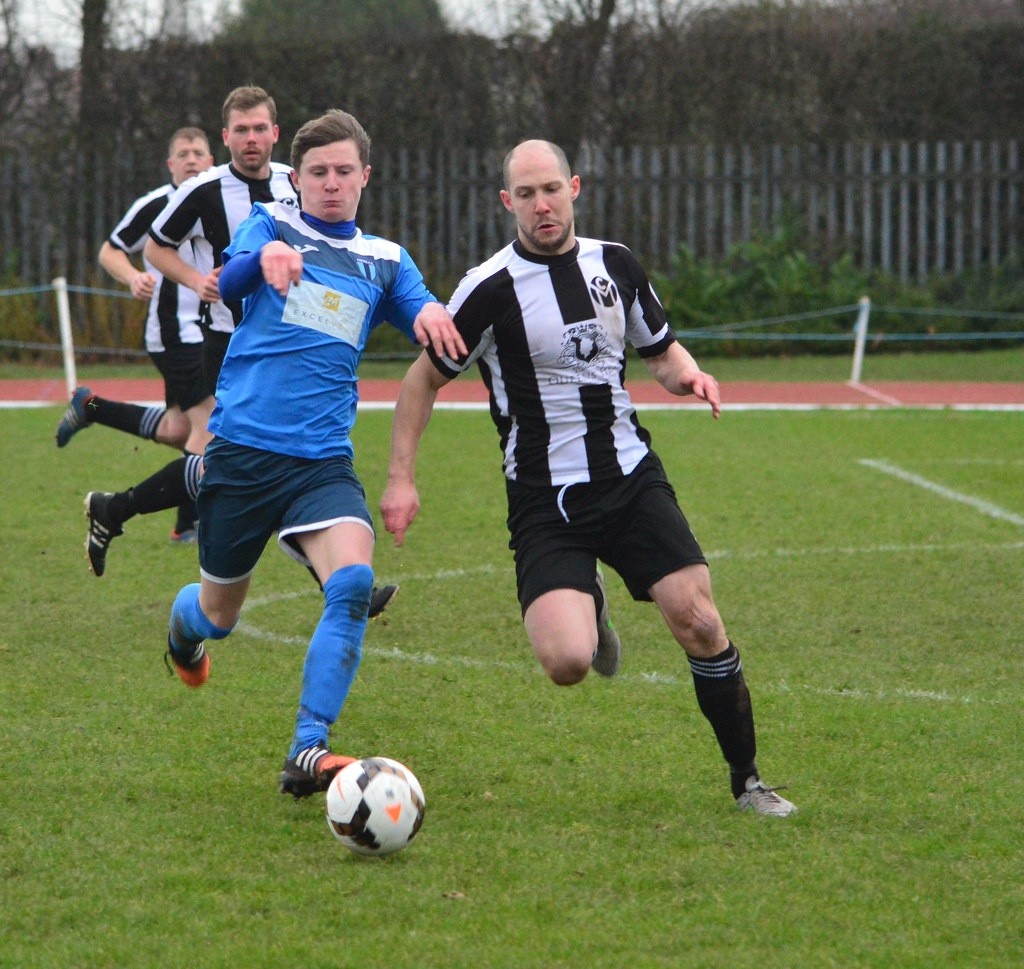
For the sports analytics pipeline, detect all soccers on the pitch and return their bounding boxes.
[325,756,426,857]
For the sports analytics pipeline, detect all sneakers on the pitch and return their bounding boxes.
[83,491,124,577]
[55,384,95,448]
[736,776,798,817]
[281,741,358,801]
[589,567,620,678]
[368,584,399,619]
[162,630,209,687]
[171,529,199,543]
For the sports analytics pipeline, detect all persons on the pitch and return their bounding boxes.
[381,138,800,821]
[164,109,470,802]
[56,81,398,623]
